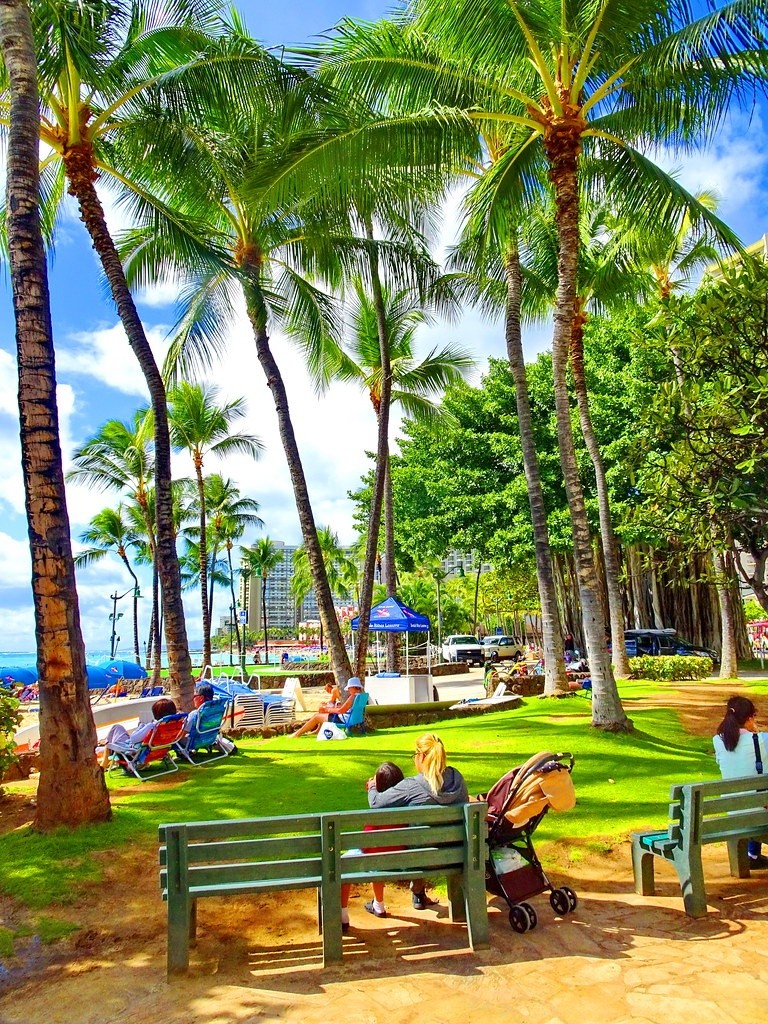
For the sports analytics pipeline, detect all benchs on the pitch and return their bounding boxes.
[158,801,490,968]
[630,773,768,918]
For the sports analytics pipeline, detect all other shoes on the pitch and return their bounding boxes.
[748,854,768,871]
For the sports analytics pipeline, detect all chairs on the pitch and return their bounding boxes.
[107,664,297,781]
[579,678,592,700]
[332,692,369,736]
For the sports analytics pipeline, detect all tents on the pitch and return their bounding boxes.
[351,597,432,677]
[197,678,285,705]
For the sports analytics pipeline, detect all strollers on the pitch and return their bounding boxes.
[465,748,579,936]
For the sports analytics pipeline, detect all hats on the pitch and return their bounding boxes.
[343,676,365,692]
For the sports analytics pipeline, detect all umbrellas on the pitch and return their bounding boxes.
[0,660,148,689]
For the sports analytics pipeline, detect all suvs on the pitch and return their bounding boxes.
[441,632,486,666]
[481,634,525,663]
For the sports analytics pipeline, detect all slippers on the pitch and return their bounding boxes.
[341,921,350,935]
[364,901,387,920]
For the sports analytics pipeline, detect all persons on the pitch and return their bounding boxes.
[341,734,468,931]
[515,633,612,687]
[254,650,289,664]
[748,631,768,650]
[713,697,767,869]
[9,682,125,699]
[100,685,214,768]
[292,677,364,737]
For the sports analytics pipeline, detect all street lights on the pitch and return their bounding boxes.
[108,584,144,661]
[432,565,466,665]
[238,564,248,676]
[224,598,241,666]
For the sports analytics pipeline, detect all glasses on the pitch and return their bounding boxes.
[191,693,204,699]
[410,753,422,761]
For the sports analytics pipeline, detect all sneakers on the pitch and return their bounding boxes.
[413,890,429,911]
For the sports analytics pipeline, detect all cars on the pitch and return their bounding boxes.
[623,628,721,665]
